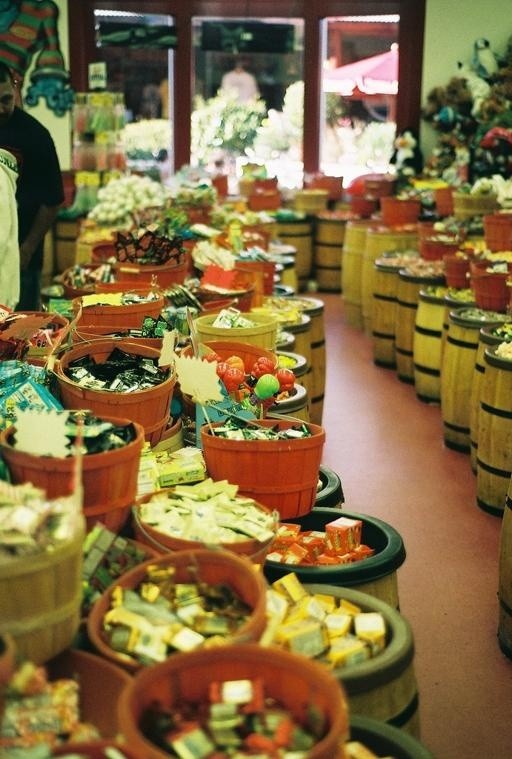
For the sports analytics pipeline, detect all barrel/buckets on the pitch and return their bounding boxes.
[348,713,430,759]
[195,311,279,348]
[443,252,476,288]
[442,305,507,452]
[315,176,344,202]
[311,465,342,508]
[496,475,512,659]
[266,579,422,740]
[414,286,473,405]
[432,187,455,219]
[0,496,83,665]
[481,212,510,248]
[178,339,280,381]
[119,643,347,759]
[485,213,512,252]
[55,217,79,270]
[199,417,325,519]
[87,549,264,658]
[268,381,311,424]
[360,224,415,319]
[262,506,405,611]
[272,306,316,391]
[395,267,445,385]
[90,237,189,287]
[273,254,299,295]
[451,188,497,225]
[317,209,352,290]
[341,220,374,303]
[272,215,313,291]
[468,258,511,312]
[379,193,418,226]
[0,406,143,542]
[476,344,512,517]
[57,337,177,449]
[470,323,512,474]
[156,416,183,441]
[370,249,422,370]
[73,282,164,336]
[0,647,134,759]
[135,487,280,567]
[420,233,462,261]
[271,241,297,256]
[295,189,328,214]
[151,431,185,452]
[279,295,325,417]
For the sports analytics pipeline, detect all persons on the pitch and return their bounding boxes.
[222,62,261,101]
[0,146,21,322]
[0,61,65,311]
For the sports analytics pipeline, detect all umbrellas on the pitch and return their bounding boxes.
[321,43,399,122]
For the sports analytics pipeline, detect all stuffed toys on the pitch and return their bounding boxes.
[390,128,423,179]
[425,34,512,214]
